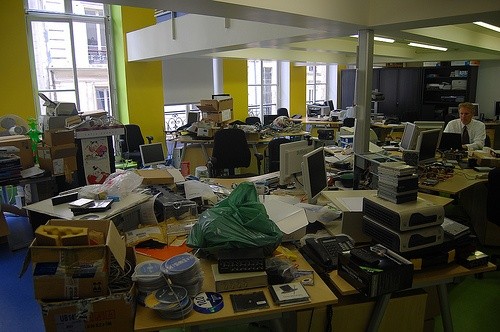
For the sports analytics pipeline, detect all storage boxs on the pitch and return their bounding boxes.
[42,129,76,146]
[17,219,126,302]
[195,99,235,125]
[38,299,135,332]
[35,146,78,178]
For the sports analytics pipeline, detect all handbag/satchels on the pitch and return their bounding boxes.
[186,182,285,257]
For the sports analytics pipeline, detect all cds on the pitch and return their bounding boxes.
[130,254,225,320]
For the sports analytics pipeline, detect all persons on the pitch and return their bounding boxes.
[444,102,486,150]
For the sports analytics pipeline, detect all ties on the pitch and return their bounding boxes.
[462,126,469,141]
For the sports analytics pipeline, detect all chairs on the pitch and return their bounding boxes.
[207,128,259,179]
[268,138,292,172]
[117,124,153,170]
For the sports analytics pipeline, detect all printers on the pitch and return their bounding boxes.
[329,109,347,121]
[307,105,329,118]
[38,92,78,116]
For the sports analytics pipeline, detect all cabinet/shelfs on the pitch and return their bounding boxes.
[340,64,479,124]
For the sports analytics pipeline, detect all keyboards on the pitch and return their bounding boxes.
[255,176,282,185]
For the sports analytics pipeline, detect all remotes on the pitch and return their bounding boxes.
[350,249,379,265]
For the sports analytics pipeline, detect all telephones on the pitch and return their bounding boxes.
[292,114,302,119]
[304,234,354,272]
[384,117,400,125]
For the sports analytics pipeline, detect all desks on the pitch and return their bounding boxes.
[0,115,500,332]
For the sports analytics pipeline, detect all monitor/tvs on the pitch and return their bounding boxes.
[278,138,313,185]
[187,112,200,124]
[399,120,463,165]
[139,142,166,169]
[301,147,329,205]
